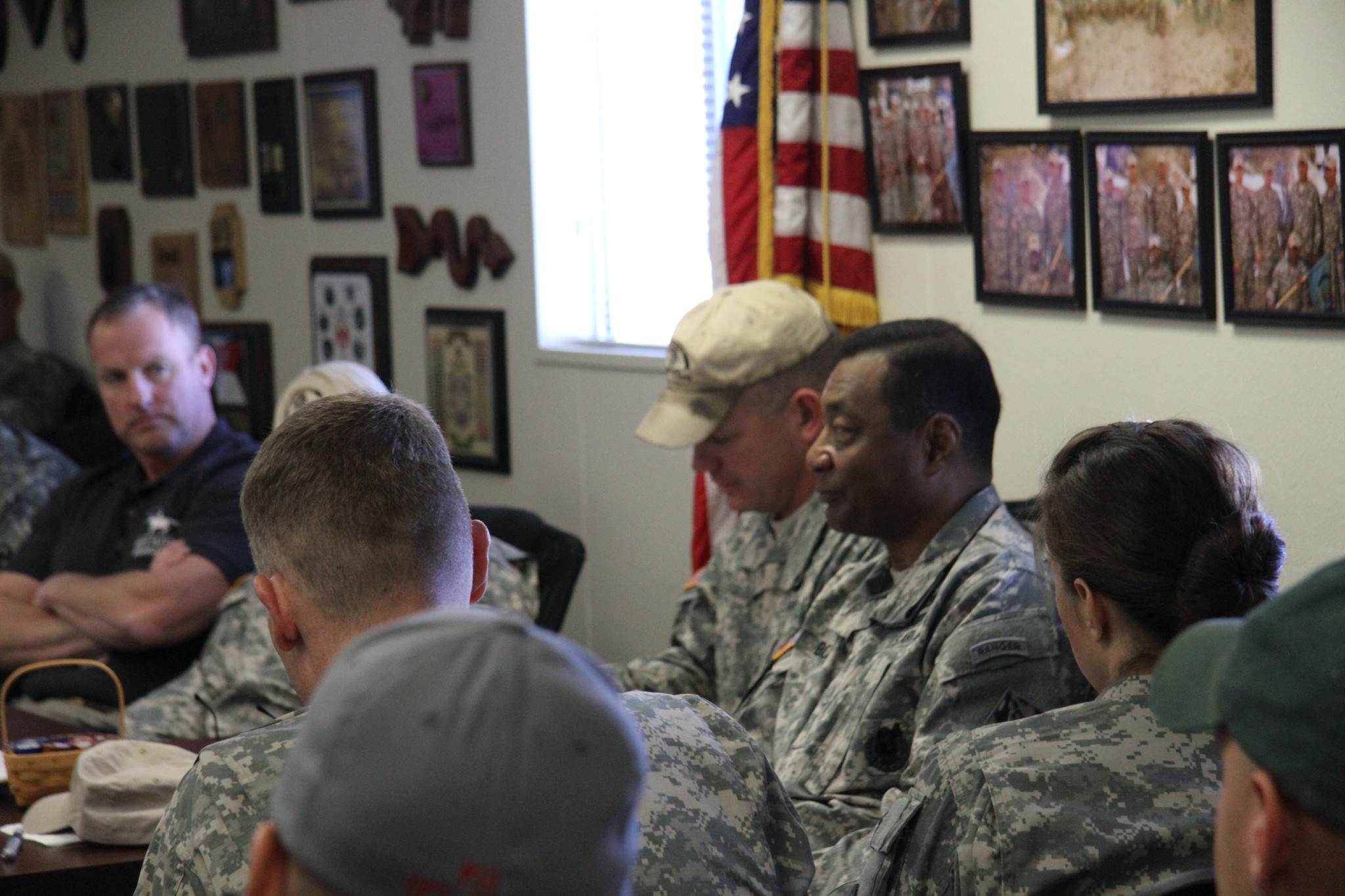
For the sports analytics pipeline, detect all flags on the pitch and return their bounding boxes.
[691,0,877,574]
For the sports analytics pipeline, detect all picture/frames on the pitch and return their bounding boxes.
[856,1,1345,332]
[3,57,511,483]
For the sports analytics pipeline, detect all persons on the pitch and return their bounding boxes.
[1150,556,1345,896]
[811,418,1282,894]
[125,397,818,896]
[584,281,842,707]
[0,249,125,568]
[0,280,263,713]
[858,57,1344,332]
[726,318,1100,861]
[107,360,535,747]
[251,609,647,896]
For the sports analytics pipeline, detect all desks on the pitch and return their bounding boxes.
[1,703,208,896]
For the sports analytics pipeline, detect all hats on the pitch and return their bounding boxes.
[992,160,1005,172]
[936,96,952,108]
[1150,557,1345,837]
[869,98,877,109]
[268,359,386,439]
[1263,162,1276,174]
[269,605,651,895]
[910,81,925,94]
[890,95,904,105]
[633,280,828,450]
[1156,157,1165,165]
[1322,157,1336,171]
[1018,173,1033,184]
[19,736,199,847]
[1103,169,1117,181]
[1232,158,1244,169]
[1181,181,1190,189]
[1126,155,1141,166]
[1297,154,1306,164]
[1048,155,1062,165]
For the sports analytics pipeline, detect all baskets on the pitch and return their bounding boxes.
[2,655,130,807]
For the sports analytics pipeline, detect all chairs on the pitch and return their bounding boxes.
[471,502,588,634]
